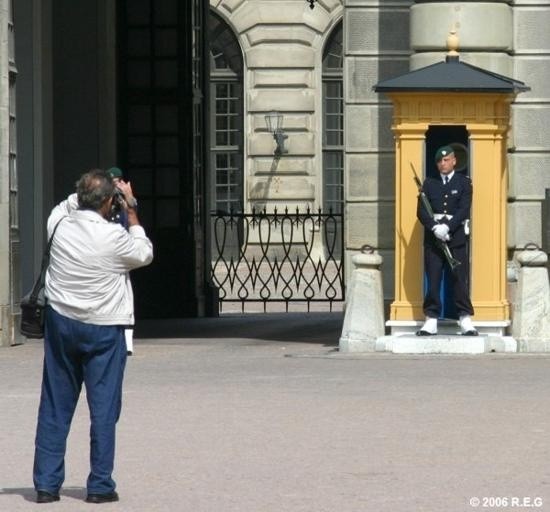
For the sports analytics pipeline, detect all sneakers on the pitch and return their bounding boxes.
[37,490,59,504]
[87,491,119,503]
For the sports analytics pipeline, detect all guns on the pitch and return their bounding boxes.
[410,163,462,271]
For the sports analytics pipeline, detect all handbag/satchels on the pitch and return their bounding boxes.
[20,287,46,338]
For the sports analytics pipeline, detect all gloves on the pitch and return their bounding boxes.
[432,223,450,242]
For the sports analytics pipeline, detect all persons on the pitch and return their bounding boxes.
[416,144,481,338]
[32,169,154,505]
[102,167,138,356]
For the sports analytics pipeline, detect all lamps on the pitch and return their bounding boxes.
[264,112,289,154]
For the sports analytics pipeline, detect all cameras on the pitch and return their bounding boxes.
[108,192,125,220]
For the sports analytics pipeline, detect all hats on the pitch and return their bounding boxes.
[435,146,453,162]
[106,167,123,177]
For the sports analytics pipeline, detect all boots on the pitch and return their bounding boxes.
[419,318,438,335]
[459,314,477,335]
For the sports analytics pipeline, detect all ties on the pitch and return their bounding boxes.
[445,176,448,183]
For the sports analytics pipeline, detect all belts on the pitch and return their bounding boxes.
[434,213,453,221]
